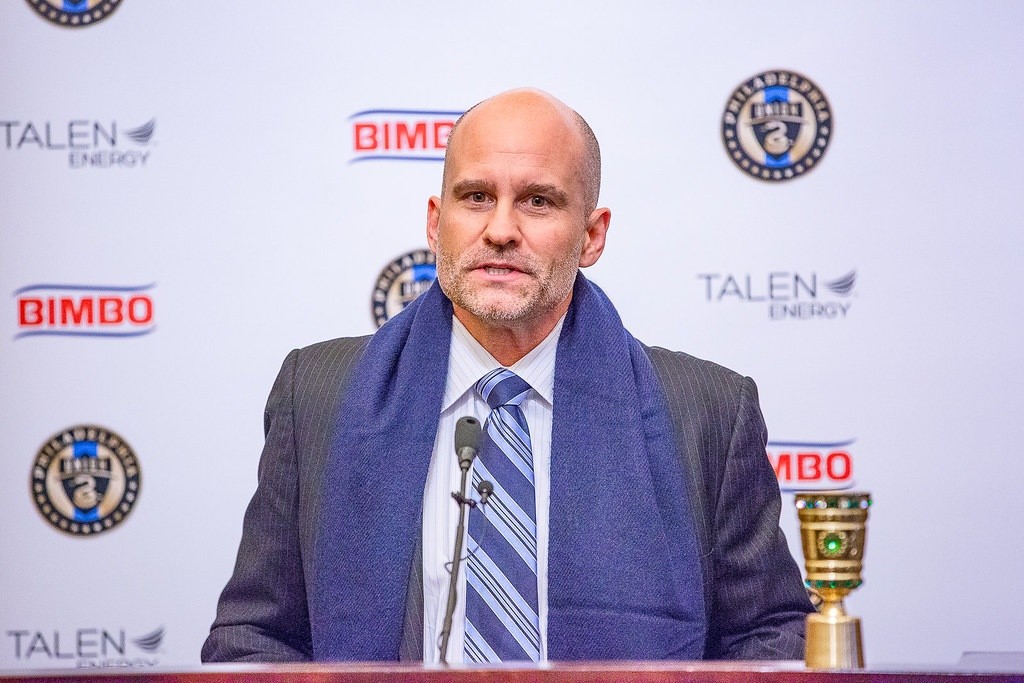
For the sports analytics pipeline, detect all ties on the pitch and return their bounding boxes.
[462,370,541,662]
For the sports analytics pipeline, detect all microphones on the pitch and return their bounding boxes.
[476,481,494,501]
[439,416,482,667]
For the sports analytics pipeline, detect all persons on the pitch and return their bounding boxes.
[200,87,816,663]
